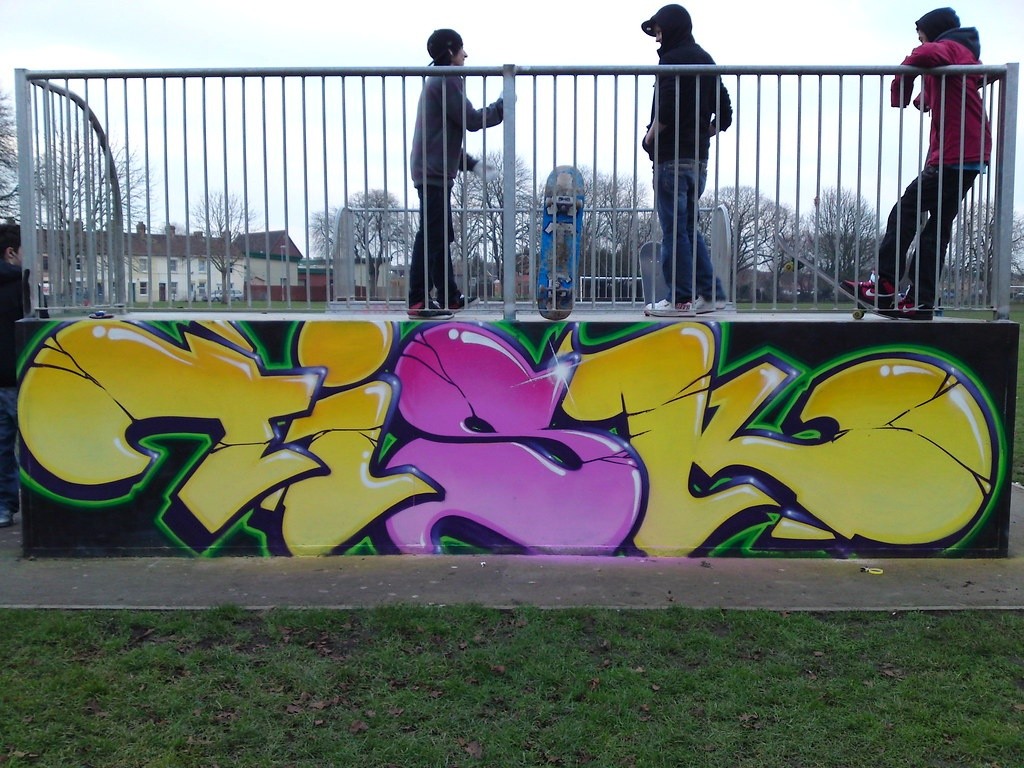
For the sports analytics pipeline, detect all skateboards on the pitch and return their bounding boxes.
[774,235,884,321]
[535,163,586,321]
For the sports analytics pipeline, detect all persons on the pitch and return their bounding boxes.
[838,5,991,319]
[639,3,735,319]
[0,223,50,530]
[406,30,519,321]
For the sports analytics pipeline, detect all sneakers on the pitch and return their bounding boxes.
[878,285,933,320]
[839,269,896,309]
[407,301,454,319]
[438,296,480,310]
[644,296,726,317]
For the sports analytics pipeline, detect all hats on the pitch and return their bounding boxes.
[915,8,960,41]
[427,29,463,66]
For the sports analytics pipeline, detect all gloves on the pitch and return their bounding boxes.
[500,89,517,103]
[474,160,498,182]
